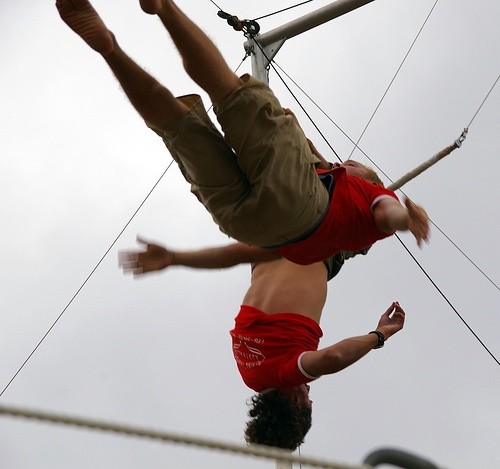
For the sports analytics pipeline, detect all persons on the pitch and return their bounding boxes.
[230,245,405,451]
[54,0,429,276]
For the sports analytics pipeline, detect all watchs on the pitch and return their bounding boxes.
[370,331,385,350]
